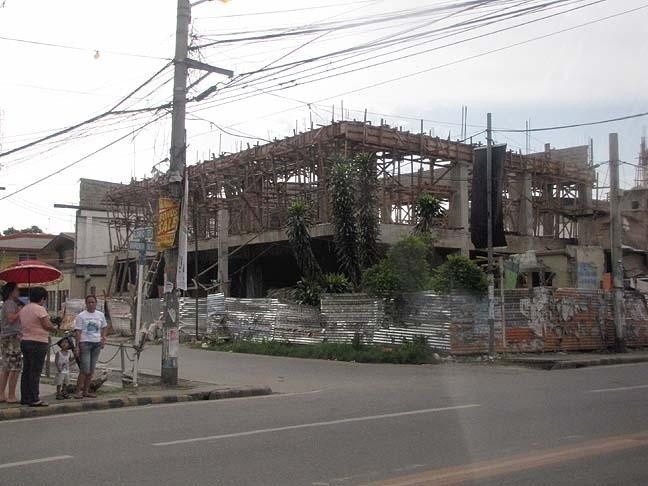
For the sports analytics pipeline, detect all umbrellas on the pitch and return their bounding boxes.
[0,257,64,303]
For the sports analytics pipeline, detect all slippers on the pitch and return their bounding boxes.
[0,398,7,403]
[7,399,20,404]
[20,400,49,407]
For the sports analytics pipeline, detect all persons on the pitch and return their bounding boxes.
[19,286,59,407]
[54,335,75,400]
[73,295,108,399]
[0,281,23,404]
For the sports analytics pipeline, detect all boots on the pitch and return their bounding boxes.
[74,375,96,399]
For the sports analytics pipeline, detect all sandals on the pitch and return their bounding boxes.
[56,393,72,400]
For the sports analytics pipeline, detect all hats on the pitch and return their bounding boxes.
[56,337,74,350]
